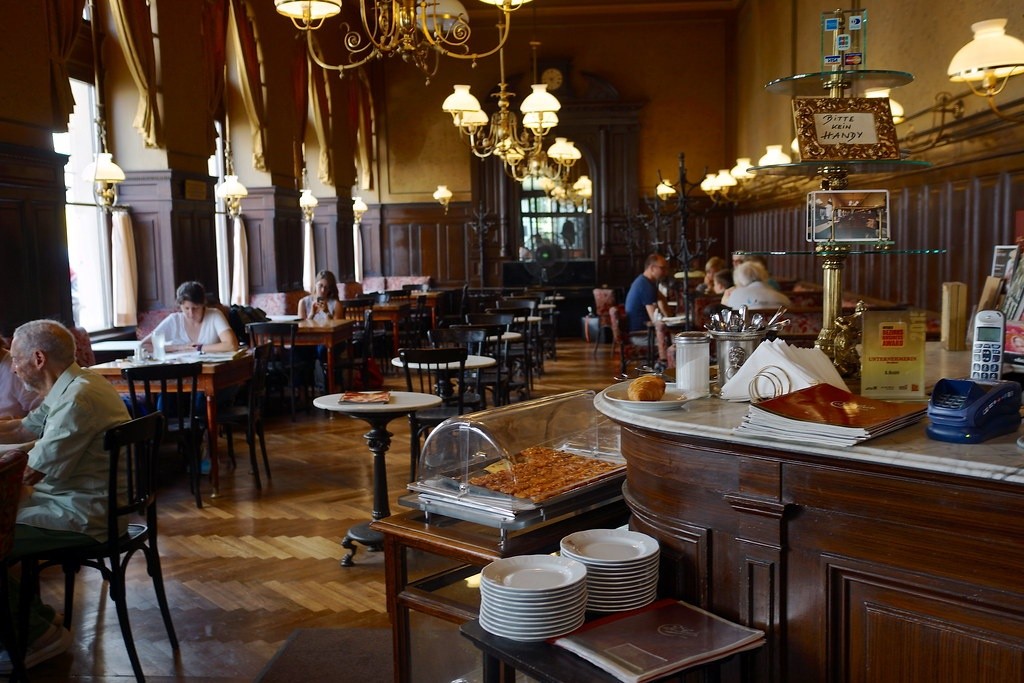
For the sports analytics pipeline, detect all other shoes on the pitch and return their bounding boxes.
[654,359,668,371]
[187,448,220,474]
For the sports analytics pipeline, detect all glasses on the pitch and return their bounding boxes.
[647,264,666,272]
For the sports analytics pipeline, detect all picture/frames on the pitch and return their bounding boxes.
[792,97,901,160]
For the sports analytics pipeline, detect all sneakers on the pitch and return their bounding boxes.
[0,612,75,674]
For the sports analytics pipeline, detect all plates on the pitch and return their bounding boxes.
[605,389,693,410]
[478,554,588,642]
[559,528,660,611]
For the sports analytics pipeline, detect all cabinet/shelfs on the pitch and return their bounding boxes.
[734,70,951,257]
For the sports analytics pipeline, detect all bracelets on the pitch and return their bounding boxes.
[197,344,203,352]
[323,308,329,314]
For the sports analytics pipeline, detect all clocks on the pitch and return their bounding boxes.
[536,55,570,98]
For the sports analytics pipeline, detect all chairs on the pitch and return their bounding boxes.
[0,277,664,683]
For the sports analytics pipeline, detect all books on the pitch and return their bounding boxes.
[546,598,767,683]
[1001,252,1024,321]
[732,382,928,447]
[338,390,391,405]
[198,348,248,361]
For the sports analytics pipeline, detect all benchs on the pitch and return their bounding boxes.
[672,275,902,336]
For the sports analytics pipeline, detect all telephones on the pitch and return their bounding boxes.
[970,310,1005,380]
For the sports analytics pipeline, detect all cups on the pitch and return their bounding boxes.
[151,331,166,360]
[712,331,769,387]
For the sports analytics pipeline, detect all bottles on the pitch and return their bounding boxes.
[675,330,711,399]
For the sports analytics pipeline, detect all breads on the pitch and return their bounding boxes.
[628,375,666,400]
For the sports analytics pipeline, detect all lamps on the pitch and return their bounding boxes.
[90,1,127,207]
[657,138,800,207]
[946,18,1024,126]
[890,87,965,156]
[274,0,599,217]
[211,21,247,217]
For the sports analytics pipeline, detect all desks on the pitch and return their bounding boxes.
[369,505,630,683]
[506,315,543,331]
[545,295,564,303]
[77,350,260,496]
[392,291,446,337]
[251,316,356,420]
[459,612,734,683]
[537,303,555,310]
[478,330,523,355]
[313,390,439,568]
[338,299,411,378]
[391,352,495,396]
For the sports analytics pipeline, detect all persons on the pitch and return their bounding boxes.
[296,270,347,407]
[826,198,832,221]
[625,254,675,370]
[139,281,238,475]
[696,250,792,310]
[0,320,136,672]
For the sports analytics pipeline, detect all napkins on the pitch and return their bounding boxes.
[720,338,852,403]
